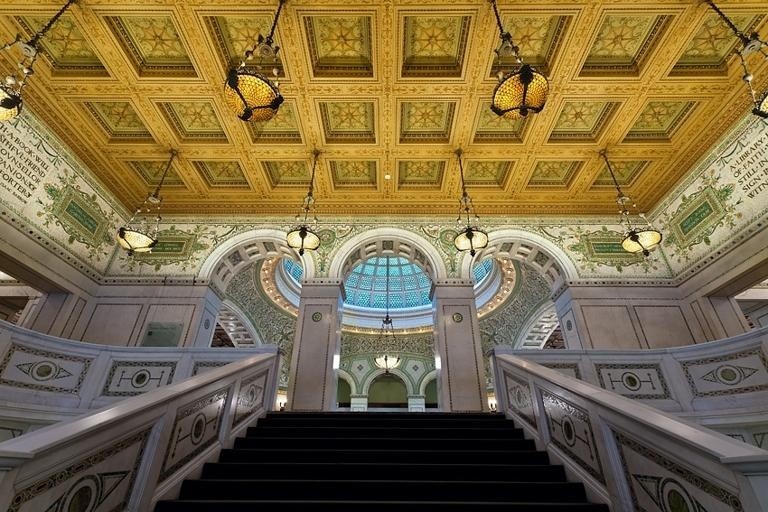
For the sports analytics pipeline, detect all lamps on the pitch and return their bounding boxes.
[0,0,76,122]
[599,148,663,257]
[116,149,178,255]
[705,0,768,117]
[490,0,549,120]
[223,0,285,123]
[374,256,402,376]
[454,148,488,256]
[286,150,321,256]
